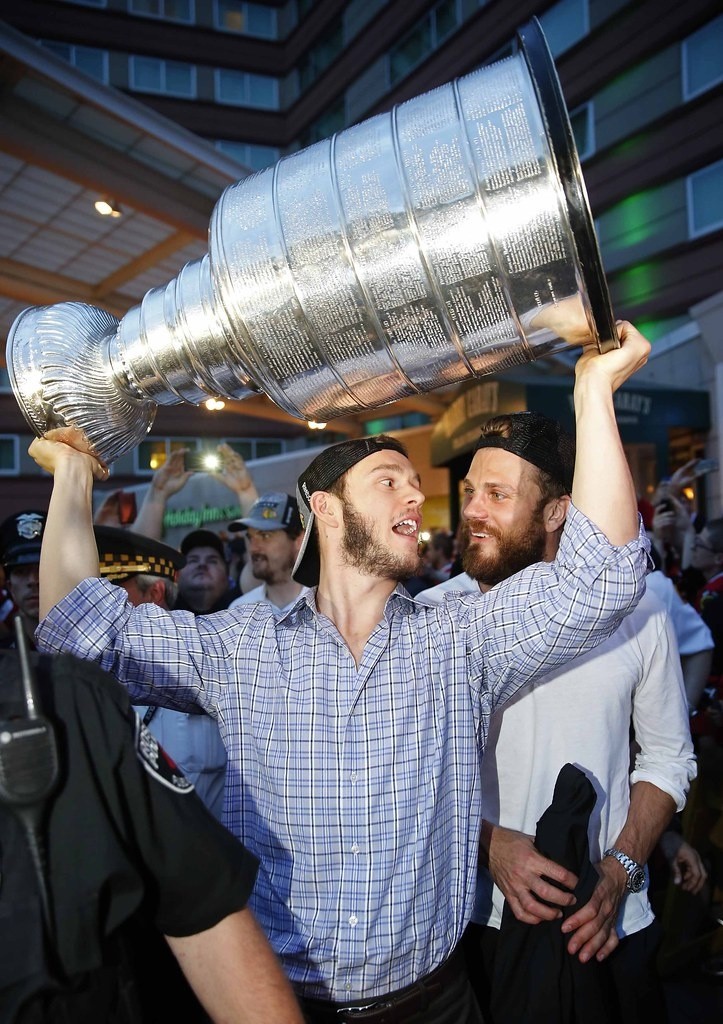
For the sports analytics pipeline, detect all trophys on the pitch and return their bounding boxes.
[6,15,622,474]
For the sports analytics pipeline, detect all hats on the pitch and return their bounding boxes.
[0,509,48,568]
[91,524,187,585]
[228,494,305,535]
[290,436,410,589]
[472,410,572,483]
[181,528,229,571]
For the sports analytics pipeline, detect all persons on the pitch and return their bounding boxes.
[27,320,652,1024]
[228,491,309,611]
[129,443,262,619]
[92,524,226,822]
[0,459,723,988]
[414,413,698,1023]
[0,648,306,1024]
[416,533,456,584]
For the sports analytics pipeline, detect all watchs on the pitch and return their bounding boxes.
[604,849,646,894]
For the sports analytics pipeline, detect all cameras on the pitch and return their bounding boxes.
[184,451,224,473]
[661,498,674,516]
[693,458,719,475]
